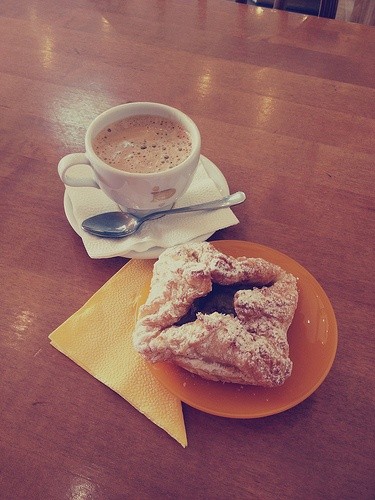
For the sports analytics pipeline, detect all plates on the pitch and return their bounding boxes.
[63,154,230,259]
[136,240,341,419]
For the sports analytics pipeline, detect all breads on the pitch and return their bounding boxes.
[132,241,299,387]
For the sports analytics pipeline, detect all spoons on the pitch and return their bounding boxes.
[81,191,246,239]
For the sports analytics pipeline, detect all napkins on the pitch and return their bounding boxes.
[49,259,188,448]
[64,160,240,260]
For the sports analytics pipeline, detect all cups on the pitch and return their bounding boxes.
[57,102,201,221]
